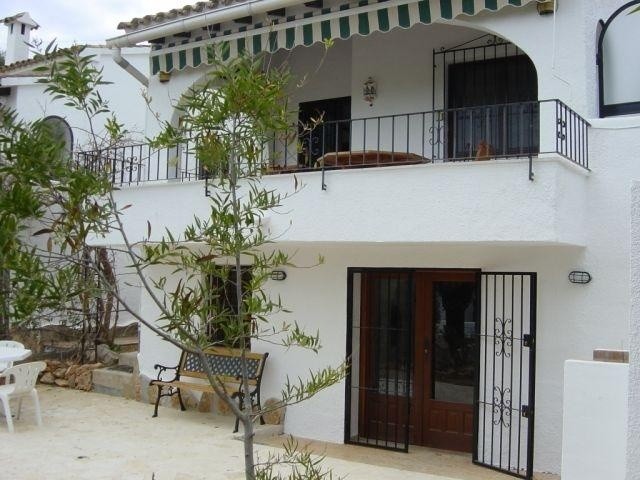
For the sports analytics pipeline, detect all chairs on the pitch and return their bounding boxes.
[0,338,47,432]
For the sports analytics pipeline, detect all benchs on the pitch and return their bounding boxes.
[150,347,267,433]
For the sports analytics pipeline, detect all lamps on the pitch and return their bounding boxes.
[271,271,286,280]
[363,75,377,107]
[569,271,590,283]
